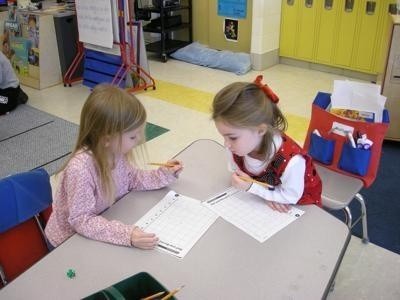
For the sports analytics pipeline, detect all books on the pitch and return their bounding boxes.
[330,107,376,123]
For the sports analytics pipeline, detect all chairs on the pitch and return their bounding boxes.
[0,168,54,286]
[301,91,389,242]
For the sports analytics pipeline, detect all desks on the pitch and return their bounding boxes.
[0,137,353,300]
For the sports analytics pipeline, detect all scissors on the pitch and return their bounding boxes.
[357,143,370,150]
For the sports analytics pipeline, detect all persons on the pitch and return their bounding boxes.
[0,51,28,116]
[212,77,326,212]
[40,81,181,260]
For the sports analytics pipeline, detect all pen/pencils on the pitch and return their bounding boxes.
[348,130,362,149]
[237,176,274,189]
[312,129,322,138]
[145,162,185,168]
[142,285,184,300]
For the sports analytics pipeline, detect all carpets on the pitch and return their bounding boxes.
[1,104,80,177]
